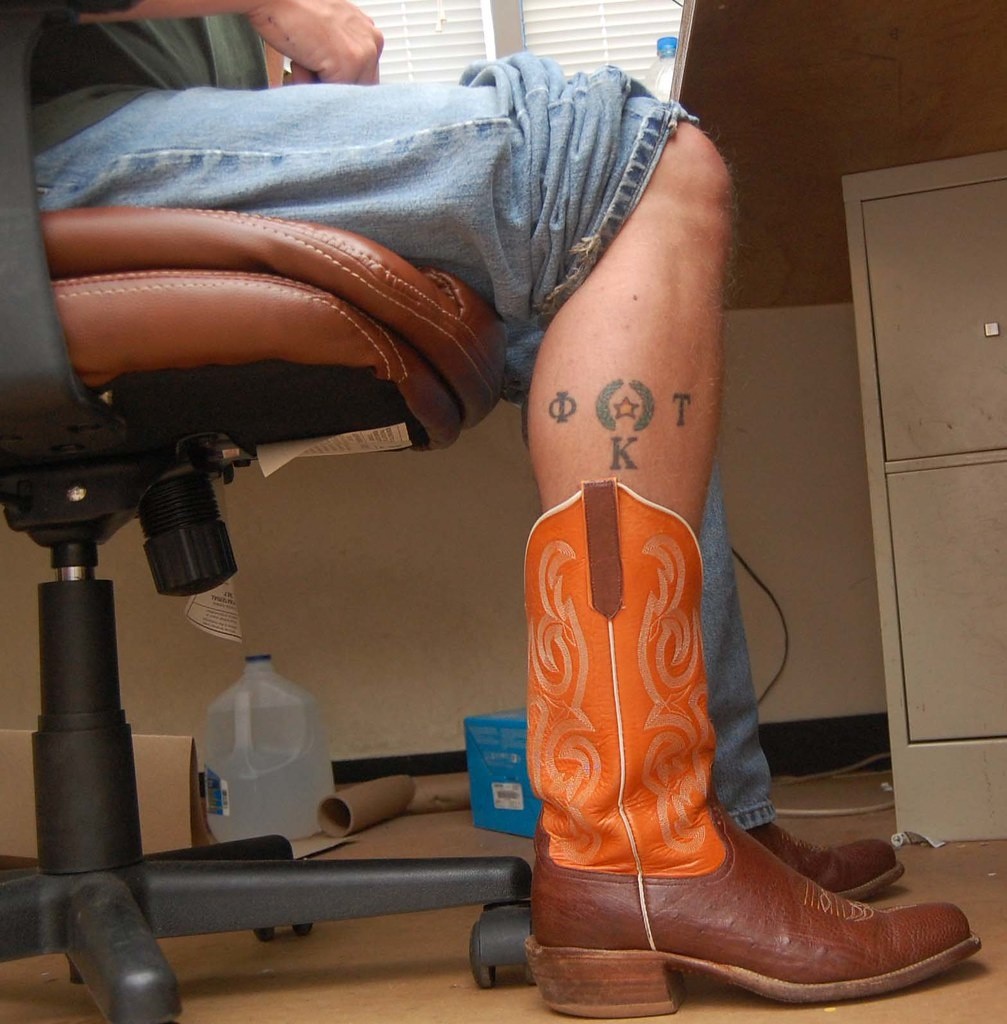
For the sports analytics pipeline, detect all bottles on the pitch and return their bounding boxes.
[201,650,334,842]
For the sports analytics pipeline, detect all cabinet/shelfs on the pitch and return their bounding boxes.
[837,148,1007,845]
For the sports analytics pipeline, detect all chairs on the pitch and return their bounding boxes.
[0,0,533,1024]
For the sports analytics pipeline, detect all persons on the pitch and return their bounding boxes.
[27,0,983,1020]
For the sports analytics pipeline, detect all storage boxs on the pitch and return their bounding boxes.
[464,706,544,839]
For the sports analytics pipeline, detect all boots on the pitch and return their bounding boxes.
[526,478,984,1019]
